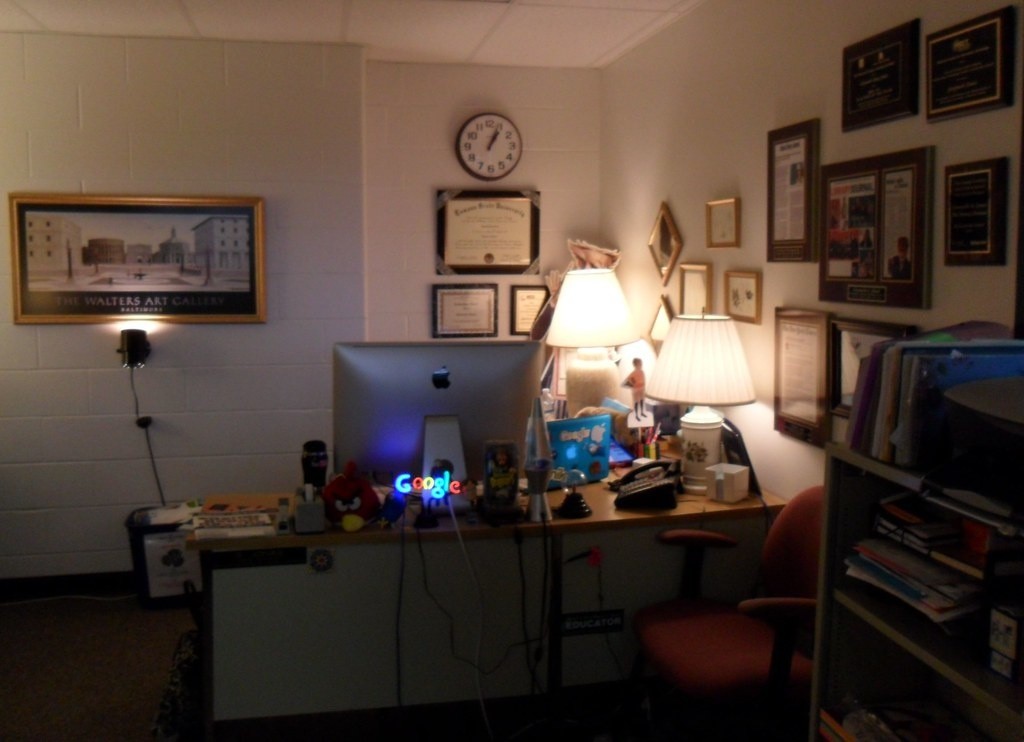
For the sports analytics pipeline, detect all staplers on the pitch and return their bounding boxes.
[275,498,289,535]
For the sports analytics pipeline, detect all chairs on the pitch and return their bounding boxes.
[630,484,823,742]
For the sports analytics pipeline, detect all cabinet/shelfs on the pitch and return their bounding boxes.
[808,440,1024,742]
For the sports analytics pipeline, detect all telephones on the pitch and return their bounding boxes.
[608,458,681,510]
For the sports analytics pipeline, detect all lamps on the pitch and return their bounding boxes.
[644,306,759,494]
[545,268,640,419]
[120,329,147,369]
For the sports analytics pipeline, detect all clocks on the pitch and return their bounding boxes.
[455,112,523,181]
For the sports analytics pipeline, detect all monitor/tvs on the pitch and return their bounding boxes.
[332,340,546,514]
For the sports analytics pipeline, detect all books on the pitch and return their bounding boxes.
[192,509,276,540]
[843,484,998,625]
[838,315,1024,472]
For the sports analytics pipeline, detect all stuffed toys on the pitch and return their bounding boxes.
[322,455,381,531]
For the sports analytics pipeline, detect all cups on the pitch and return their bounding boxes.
[633,442,659,459]
[707,463,750,503]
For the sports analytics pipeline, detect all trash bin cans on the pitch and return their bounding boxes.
[125,506,209,611]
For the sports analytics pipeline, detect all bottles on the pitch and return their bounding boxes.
[301,440,328,495]
[275,497,290,533]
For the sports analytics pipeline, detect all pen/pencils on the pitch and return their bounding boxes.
[646,422,661,444]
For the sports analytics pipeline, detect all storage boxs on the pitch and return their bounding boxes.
[705,463,750,504]
[124,506,186,613]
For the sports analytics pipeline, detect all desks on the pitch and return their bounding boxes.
[185,469,790,742]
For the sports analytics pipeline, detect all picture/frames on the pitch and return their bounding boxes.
[647,294,673,358]
[818,144,936,310]
[829,320,921,418]
[648,201,683,286]
[679,263,713,315]
[766,117,820,263]
[841,17,921,133]
[724,272,762,326]
[7,192,267,325]
[431,283,499,339]
[705,196,742,249]
[510,285,551,335]
[944,156,1010,266]
[926,4,1017,123]
[773,306,835,449]
[436,189,540,275]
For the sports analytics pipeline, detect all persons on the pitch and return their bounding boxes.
[890,236,912,280]
[828,196,872,278]
[622,358,647,422]
